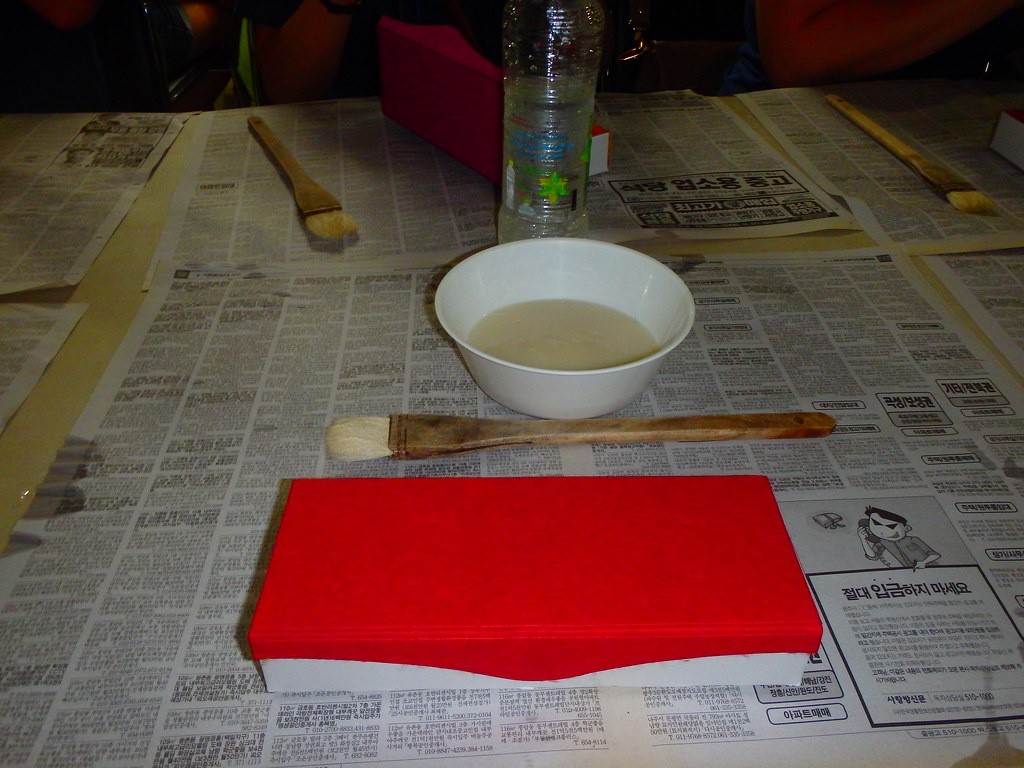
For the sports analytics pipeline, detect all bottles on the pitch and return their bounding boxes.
[496,0,604,245]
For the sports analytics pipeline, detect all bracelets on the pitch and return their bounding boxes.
[321,0,361,16]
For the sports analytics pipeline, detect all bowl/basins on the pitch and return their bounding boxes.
[433,237,696,421]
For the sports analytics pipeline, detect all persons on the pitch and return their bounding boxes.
[0,0,507,114]
[716,0,1024,99]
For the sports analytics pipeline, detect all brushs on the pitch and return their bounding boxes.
[824,93,993,214]
[323,410,835,464]
[246,115,358,239]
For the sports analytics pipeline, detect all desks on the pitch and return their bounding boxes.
[0,87,1024,768]
